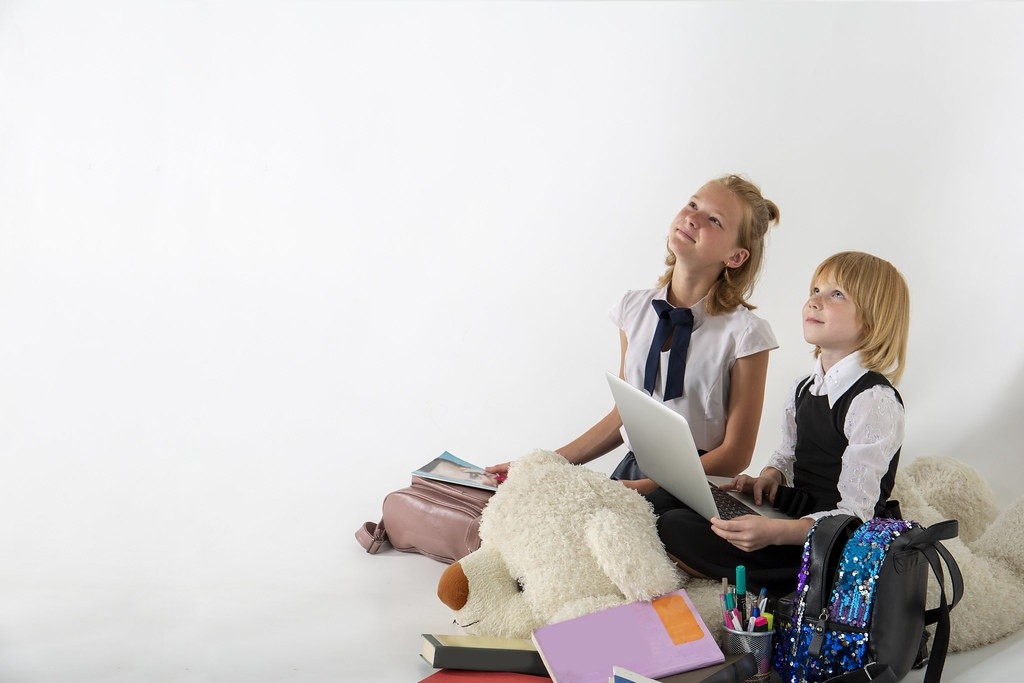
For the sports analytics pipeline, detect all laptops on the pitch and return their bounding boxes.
[606,372,798,527]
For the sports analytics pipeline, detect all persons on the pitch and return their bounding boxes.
[644,250,912,595]
[419,458,498,487]
[484,172,780,494]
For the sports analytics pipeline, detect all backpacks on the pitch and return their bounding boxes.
[771,500,964,683]
[355,475,496,565]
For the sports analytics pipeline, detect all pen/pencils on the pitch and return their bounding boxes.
[719,565,775,676]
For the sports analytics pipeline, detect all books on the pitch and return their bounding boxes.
[530,587,727,682]
[410,451,497,492]
[420,633,549,676]
[417,668,553,683]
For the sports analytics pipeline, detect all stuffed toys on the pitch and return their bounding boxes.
[429,449,1024,657]
